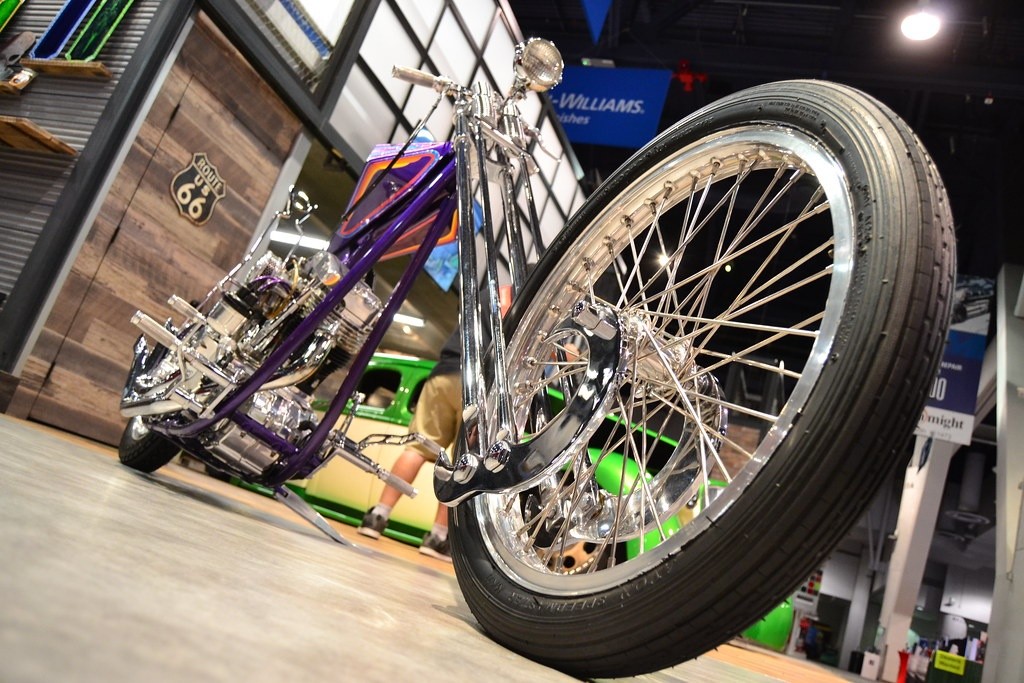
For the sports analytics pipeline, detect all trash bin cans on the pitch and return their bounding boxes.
[859,650,881,681]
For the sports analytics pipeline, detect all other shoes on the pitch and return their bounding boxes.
[358,507,390,539]
[419,532,453,563]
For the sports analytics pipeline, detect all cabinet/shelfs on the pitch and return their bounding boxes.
[0,57,113,156]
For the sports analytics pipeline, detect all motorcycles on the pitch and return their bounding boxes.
[115,32,961,681]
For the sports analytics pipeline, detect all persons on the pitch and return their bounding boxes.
[359,263,579,562]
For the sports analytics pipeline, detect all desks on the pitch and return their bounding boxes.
[926,651,984,683]
